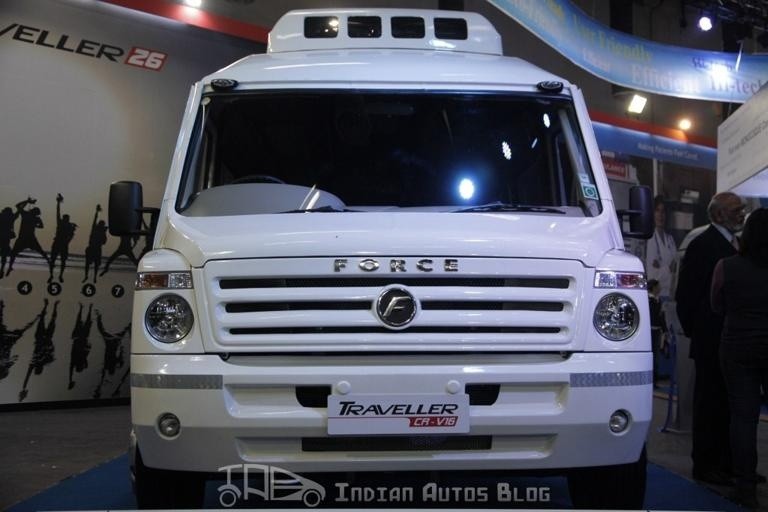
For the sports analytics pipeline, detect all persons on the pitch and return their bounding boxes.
[675,190,767,490]
[713,206,768,510]
[646,278,669,390]
[642,195,680,345]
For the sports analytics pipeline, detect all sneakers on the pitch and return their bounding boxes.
[691,464,768,509]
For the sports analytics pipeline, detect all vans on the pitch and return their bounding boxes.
[104,7,658,508]
